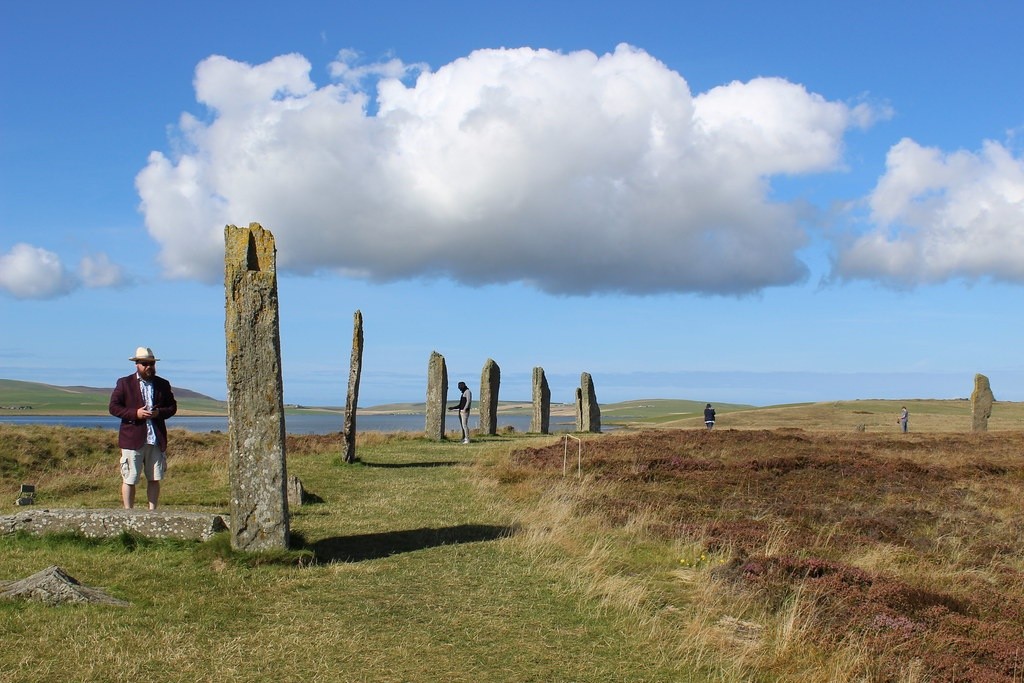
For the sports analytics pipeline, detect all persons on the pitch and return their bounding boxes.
[896,416,900,424]
[448,381,473,445]
[108,346,178,511]
[703,403,716,430]
[900,406,909,433]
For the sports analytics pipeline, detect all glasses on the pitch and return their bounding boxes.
[137,360,155,366]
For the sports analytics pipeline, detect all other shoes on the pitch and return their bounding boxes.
[459,438,466,441]
[462,439,470,444]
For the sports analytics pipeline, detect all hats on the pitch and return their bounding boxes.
[128,346,160,361]
